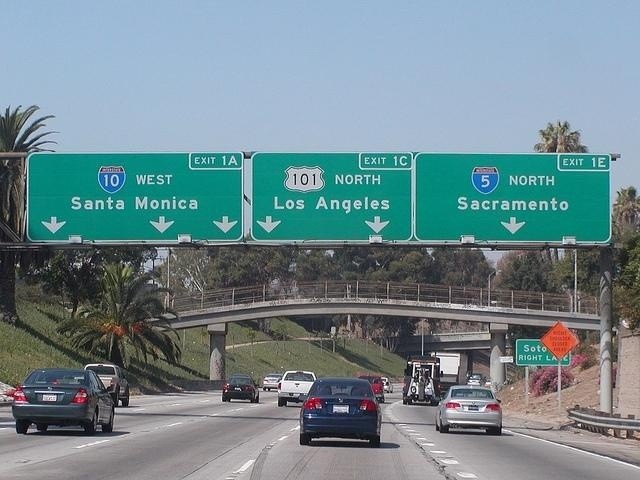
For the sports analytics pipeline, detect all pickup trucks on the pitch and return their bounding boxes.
[278,370,317,407]
[74,364,129,407]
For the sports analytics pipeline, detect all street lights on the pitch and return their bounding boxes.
[418,319,427,355]
[487,270,502,306]
[563,288,573,313]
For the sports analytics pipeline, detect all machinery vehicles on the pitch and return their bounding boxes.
[403,355,443,407]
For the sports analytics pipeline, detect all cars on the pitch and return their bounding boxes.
[263,374,283,391]
[435,385,502,436]
[472,293,504,307]
[297,377,385,448]
[381,377,393,393]
[467,373,491,386]
[12,368,116,436]
[222,376,259,403]
[357,373,389,403]
[233,374,256,385]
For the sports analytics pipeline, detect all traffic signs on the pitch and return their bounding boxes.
[26,150,243,245]
[413,153,612,244]
[252,151,414,244]
[516,338,571,367]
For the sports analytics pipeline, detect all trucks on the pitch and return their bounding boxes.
[431,352,468,398]
